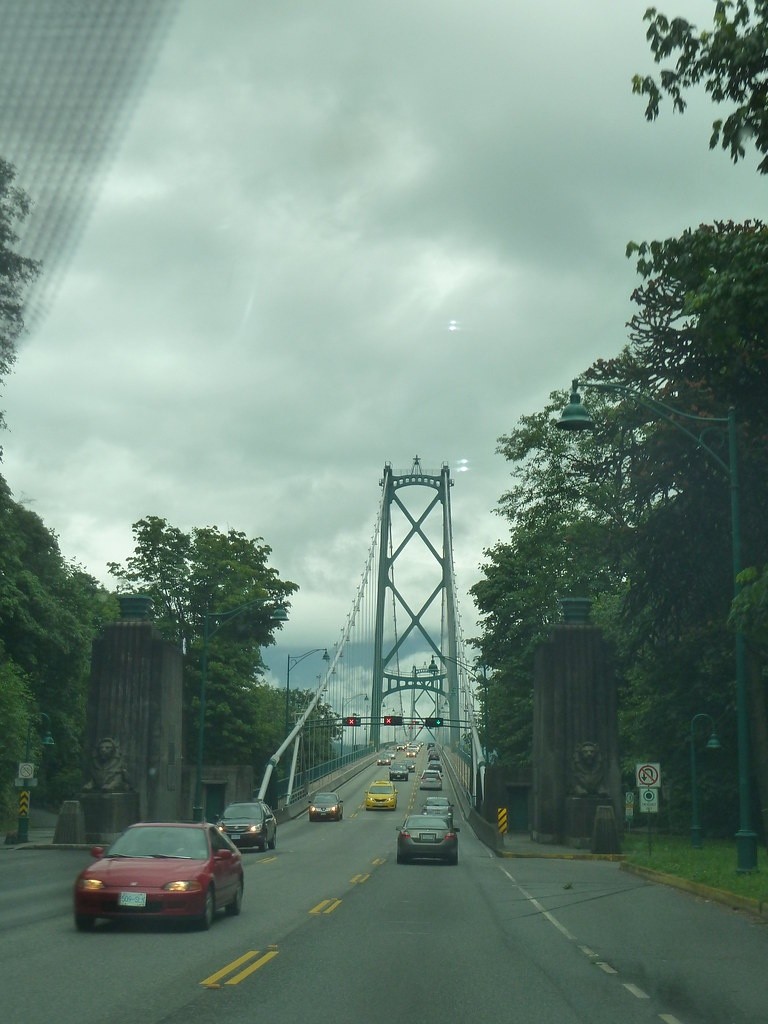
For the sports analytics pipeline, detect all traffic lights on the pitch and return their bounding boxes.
[347,717,355,726]
[425,718,443,727]
[384,716,403,726]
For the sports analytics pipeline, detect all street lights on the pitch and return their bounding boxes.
[556,378,761,876]
[191,597,293,830]
[340,693,369,757]
[428,654,490,764]
[689,713,720,848]
[381,708,395,732]
[366,701,386,747]
[17,712,55,842]
[284,648,332,777]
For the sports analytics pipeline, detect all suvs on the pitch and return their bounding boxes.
[215,801,278,850]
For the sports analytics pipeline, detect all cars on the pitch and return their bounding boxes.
[307,791,345,822]
[364,781,400,811]
[74,823,245,934]
[395,815,461,866]
[419,797,455,824]
[375,740,444,791]
